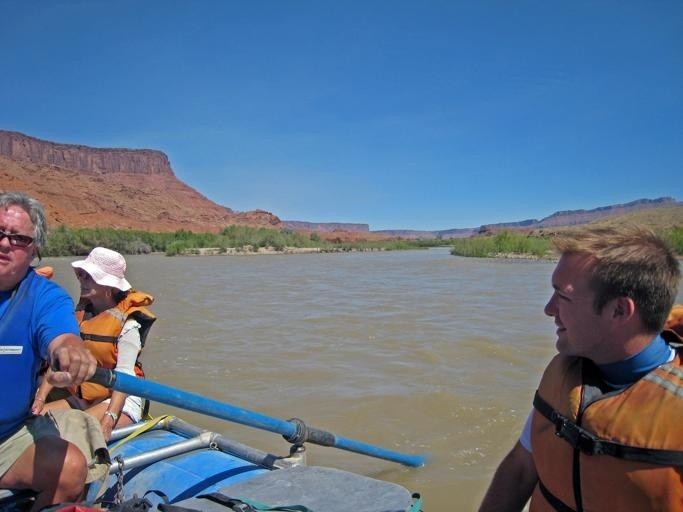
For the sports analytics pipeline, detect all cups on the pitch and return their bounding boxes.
[75,271,91,281]
[0,229,35,247]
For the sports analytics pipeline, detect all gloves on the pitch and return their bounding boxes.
[0,415,421,512]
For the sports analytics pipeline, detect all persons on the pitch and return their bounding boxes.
[478,223,682,511]
[26,247,156,442]
[0,191,99,511]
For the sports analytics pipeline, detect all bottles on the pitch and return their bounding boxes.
[72,247,132,292]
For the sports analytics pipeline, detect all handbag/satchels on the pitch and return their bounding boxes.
[34,397,45,402]
[104,410,117,424]
